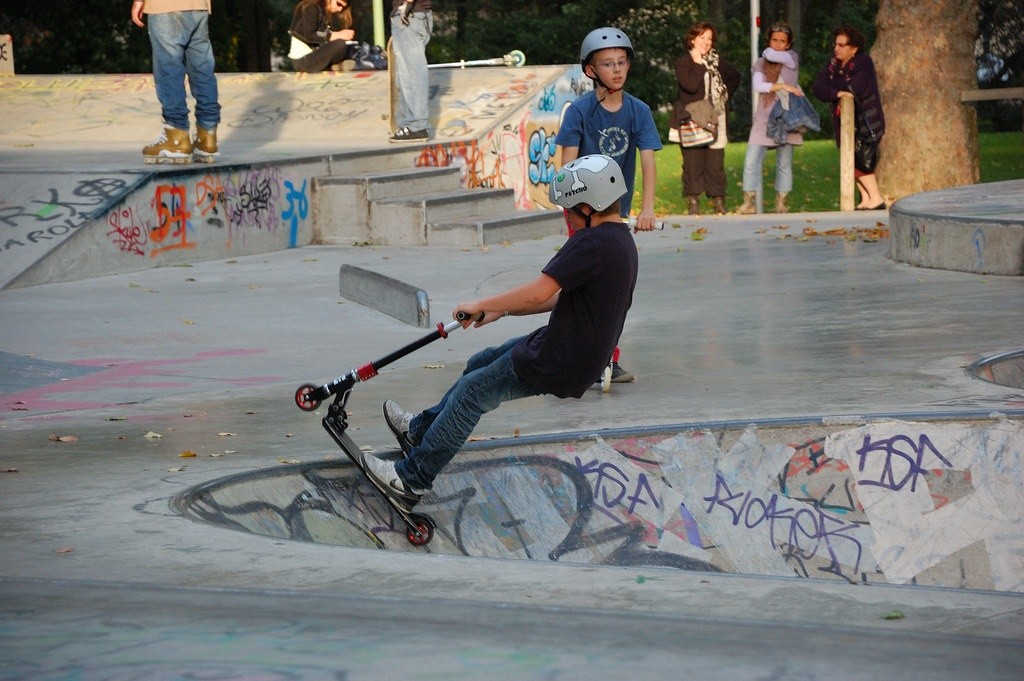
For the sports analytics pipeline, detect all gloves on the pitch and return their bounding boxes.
[400,1,414,26]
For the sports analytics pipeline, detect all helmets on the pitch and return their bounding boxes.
[581,27,633,71]
[548,154,628,212]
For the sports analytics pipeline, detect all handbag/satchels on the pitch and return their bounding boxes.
[782,85,813,134]
[679,118,714,148]
[853,132,877,172]
[345,40,388,70]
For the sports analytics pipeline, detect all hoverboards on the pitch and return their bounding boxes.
[427,50,525,69]
[294,306,486,545]
[597,217,664,392]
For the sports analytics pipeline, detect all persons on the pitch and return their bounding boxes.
[734,23,805,214]
[389,0,433,143]
[810,25,885,211]
[554,27,663,382]
[667,21,740,216]
[131,0,222,158]
[287,0,358,73]
[361,154,639,517]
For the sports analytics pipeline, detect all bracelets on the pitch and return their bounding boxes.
[503,310,508,317]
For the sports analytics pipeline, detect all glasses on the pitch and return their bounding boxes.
[834,43,849,47]
[589,60,629,70]
[336,2,347,11]
[772,26,790,33]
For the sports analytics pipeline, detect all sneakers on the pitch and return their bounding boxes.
[608,362,635,383]
[389,126,428,142]
[358,451,424,513]
[384,399,420,459]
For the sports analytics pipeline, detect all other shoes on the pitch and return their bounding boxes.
[855,201,886,209]
[331,59,356,71]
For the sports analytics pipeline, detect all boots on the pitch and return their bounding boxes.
[190,120,220,164]
[734,190,756,214]
[145,123,191,163]
[776,191,788,214]
[686,194,700,216]
[712,195,729,215]
